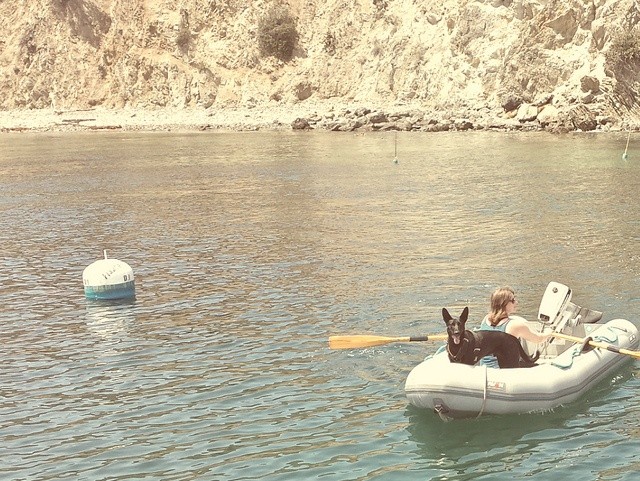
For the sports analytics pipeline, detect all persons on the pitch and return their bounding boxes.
[473,285,556,368]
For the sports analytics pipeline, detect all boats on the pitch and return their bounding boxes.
[403,315,639,416]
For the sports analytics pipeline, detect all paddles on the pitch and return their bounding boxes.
[550,332,640,358]
[329,334,449,349]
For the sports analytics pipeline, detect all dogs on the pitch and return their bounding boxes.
[439,307,539,368]
